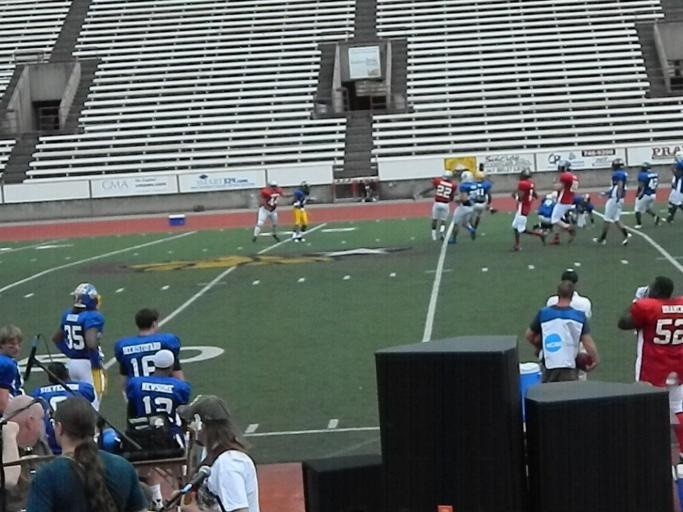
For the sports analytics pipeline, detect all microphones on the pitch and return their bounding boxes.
[181,464,211,495]
[22,333,43,382]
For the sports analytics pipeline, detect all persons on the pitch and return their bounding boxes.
[0,394,55,512]
[536,190,559,245]
[411,170,457,241]
[52,282,106,433]
[251,181,294,243]
[592,158,632,246]
[531,194,595,232]
[114,307,184,404]
[447,170,477,245]
[121,349,192,452]
[469,170,492,241]
[175,393,260,512]
[634,161,660,229]
[0,323,27,417]
[661,152,683,224]
[508,167,546,252]
[549,160,578,247]
[26,396,148,512]
[525,280,600,384]
[292,180,317,244]
[544,267,593,383]
[618,275,683,464]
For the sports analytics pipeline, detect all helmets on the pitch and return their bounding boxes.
[612,158,624,166]
[641,161,651,169]
[74,283,97,308]
[676,151,683,162]
[521,168,531,177]
[559,159,571,171]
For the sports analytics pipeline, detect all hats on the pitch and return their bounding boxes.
[177,394,230,422]
[154,350,174,368]
[475,171,485,182]
[455,164,468,170]
[461,171,473,183]
[561,269,578,283]
[442,170,452,180]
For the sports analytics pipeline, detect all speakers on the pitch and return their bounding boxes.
[375,335,526,511]
[529,380,677,511]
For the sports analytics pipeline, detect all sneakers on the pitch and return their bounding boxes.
[592,238,606,245]
[622,233,632,245]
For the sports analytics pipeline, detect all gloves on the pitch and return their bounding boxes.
[635,287,648,299]
[646,285,649,296]
